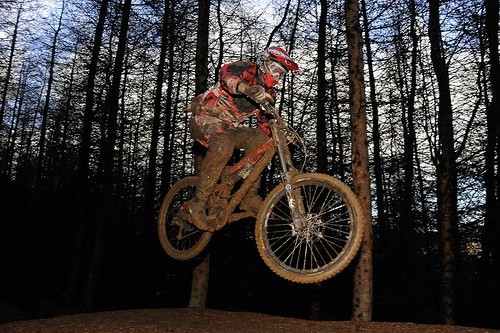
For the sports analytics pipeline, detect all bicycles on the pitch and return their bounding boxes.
[159,87,365,283]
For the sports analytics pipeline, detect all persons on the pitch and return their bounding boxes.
[186,46,300,231]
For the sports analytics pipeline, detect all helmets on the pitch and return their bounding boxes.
[255,46,298,88]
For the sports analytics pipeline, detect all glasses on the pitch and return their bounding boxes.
[268,62,286,81]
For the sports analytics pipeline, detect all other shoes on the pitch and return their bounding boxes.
[186,196,209,231]
[240,194,275,218]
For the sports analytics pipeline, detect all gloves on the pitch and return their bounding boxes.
[277,117,287,129]
[244,85,266,104]
